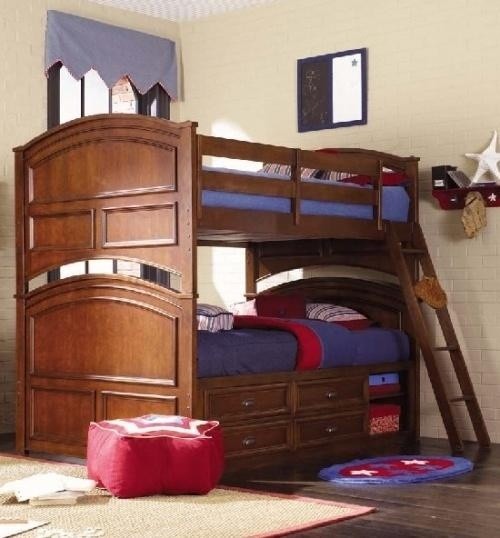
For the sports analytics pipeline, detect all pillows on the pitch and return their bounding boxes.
[196,283,375,335]
[257,161,409,188]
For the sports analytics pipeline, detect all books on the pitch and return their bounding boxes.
[5,471,99,506]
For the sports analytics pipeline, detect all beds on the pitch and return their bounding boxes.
[8,110,424,471]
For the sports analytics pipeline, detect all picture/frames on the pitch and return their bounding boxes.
[297,47,368,132]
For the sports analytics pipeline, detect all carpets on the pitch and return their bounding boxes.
[318,455,476,485]
[2,454,381,538]
